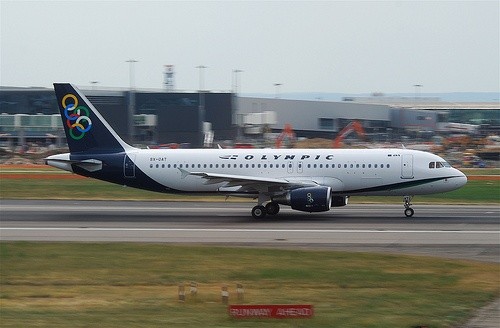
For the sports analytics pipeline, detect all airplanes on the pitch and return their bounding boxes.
[45,83,468,218]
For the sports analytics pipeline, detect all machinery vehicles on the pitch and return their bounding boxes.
[430,134,500,168]
[274,122,296,149]
[333,120,366,148]
